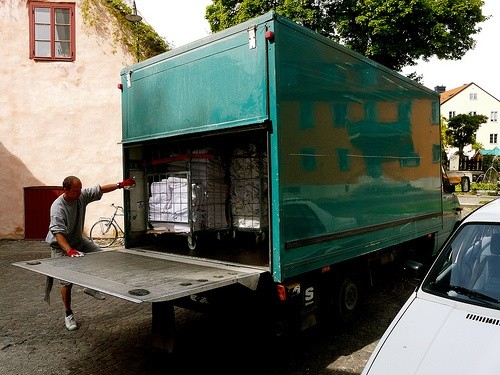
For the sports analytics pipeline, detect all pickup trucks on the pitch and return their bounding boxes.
[441,162,474,194]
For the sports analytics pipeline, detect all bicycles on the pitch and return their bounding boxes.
[89,202,125,248]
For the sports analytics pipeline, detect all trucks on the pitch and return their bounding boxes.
[9,5,463,356]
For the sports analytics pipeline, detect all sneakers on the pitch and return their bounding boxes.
[84,288,105,300]
[65,313,77,330]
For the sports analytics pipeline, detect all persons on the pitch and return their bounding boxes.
[45,176,136,330]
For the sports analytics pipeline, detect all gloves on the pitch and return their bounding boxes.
[120,177,136,190]
[67,249,84,258]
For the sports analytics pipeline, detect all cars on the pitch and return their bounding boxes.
[358,196,500,375]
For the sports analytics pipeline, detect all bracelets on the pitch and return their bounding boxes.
[117,183,120,189]
